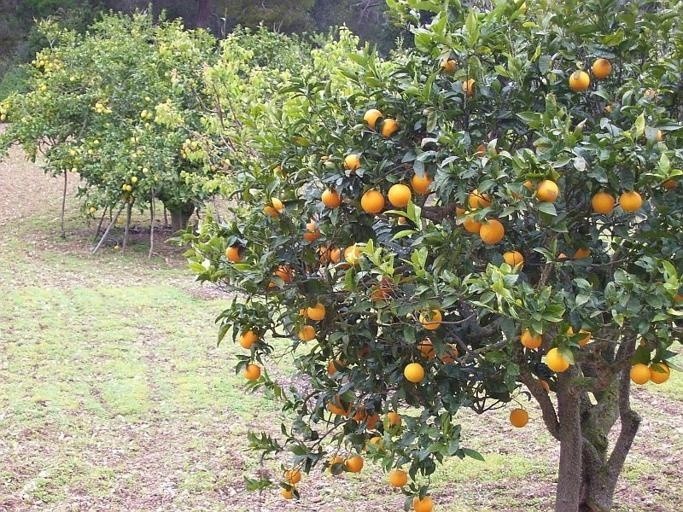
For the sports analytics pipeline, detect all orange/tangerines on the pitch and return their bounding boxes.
[0,55,198,218]
[224,53,681,512]
